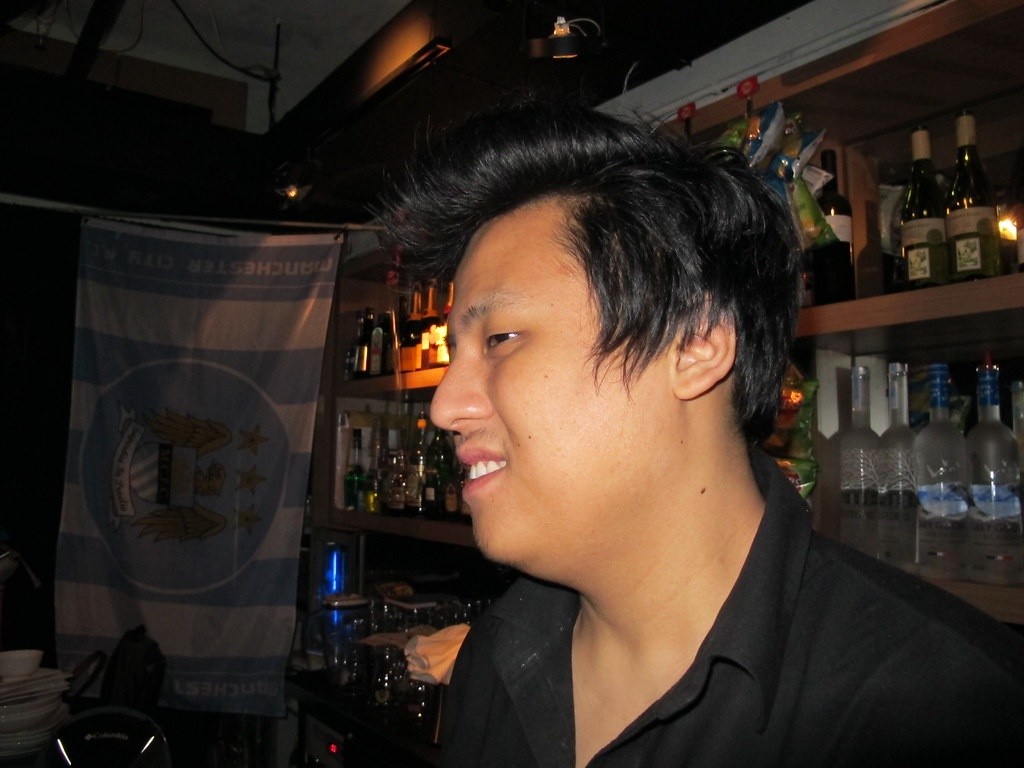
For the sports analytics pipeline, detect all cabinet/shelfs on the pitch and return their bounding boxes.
[299,0,1024,768]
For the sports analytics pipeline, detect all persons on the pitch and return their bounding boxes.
[362,104,1024,768]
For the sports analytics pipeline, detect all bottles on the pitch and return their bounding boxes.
[810,150,854,308]
[899,110,1002,290]
[341,276,455,382]
[344,408,483,524]
[840,362,1024,586]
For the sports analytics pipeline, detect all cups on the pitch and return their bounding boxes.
[302,581,442,711]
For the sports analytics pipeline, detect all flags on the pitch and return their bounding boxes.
[55,219,343,715]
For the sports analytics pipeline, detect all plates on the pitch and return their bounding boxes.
[0,668,73,756]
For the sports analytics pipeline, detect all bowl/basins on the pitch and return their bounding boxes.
[0,649,43,677]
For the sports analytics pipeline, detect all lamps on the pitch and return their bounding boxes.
[548,16,607,59]
